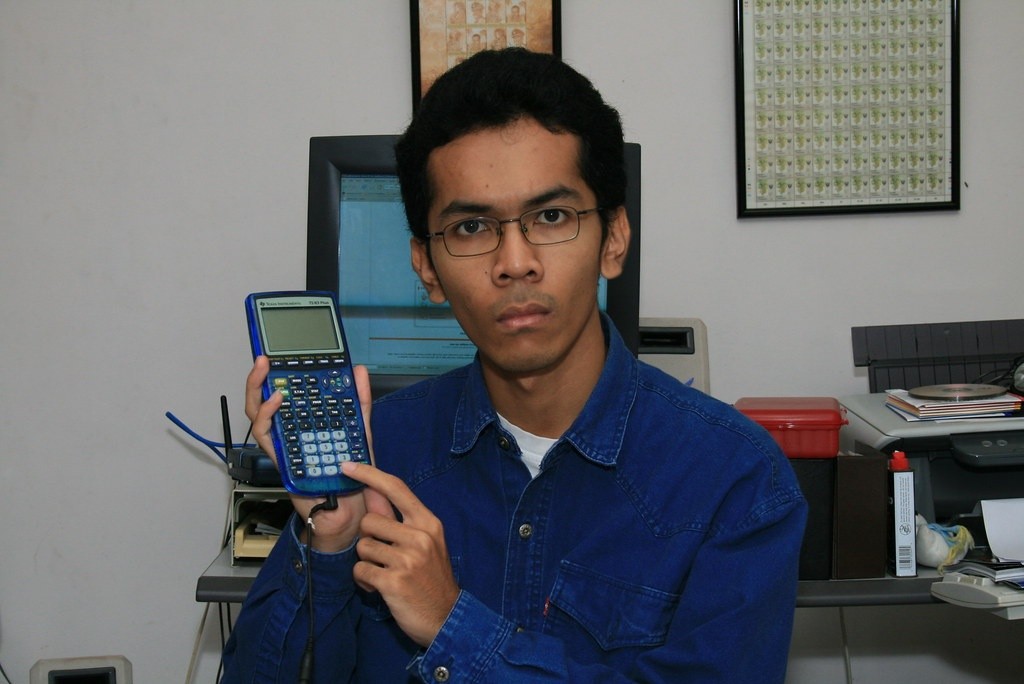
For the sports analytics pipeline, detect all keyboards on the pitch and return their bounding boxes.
[931,572,1024,621]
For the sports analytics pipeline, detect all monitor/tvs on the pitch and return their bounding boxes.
[307,134,641,401]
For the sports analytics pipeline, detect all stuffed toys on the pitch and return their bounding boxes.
[914,514,975,573]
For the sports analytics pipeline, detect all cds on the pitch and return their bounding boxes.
[908,383,1008,402]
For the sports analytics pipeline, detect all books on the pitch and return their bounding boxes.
[942,560,1024,588]
[885,392,1024,422]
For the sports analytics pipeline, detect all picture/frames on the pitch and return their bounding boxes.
[409,0,562,120]
[731,0,965,221]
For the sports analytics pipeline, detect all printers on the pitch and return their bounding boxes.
[838,318,1024,548]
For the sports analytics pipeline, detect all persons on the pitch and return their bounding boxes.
[450,2,525,63]
[219,46,807,684]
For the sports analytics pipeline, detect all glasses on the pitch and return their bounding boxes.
[423,205,605,257]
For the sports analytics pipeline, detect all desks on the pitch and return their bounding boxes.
[192,532,961,611]
